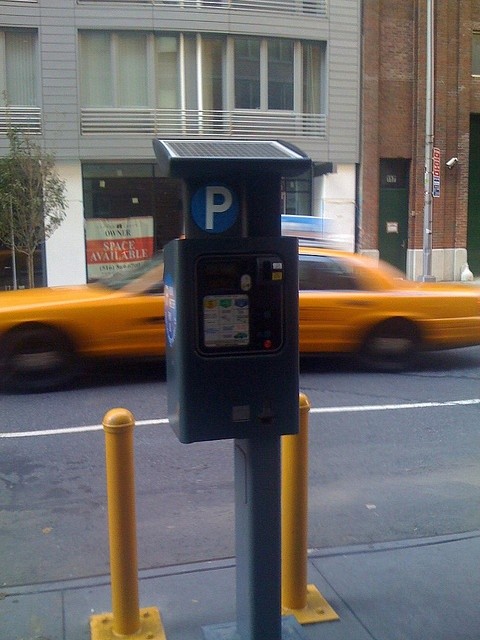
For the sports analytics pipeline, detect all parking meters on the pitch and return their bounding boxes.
[151,136,312,636]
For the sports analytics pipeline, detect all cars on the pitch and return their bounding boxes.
[1,234,480,393]
